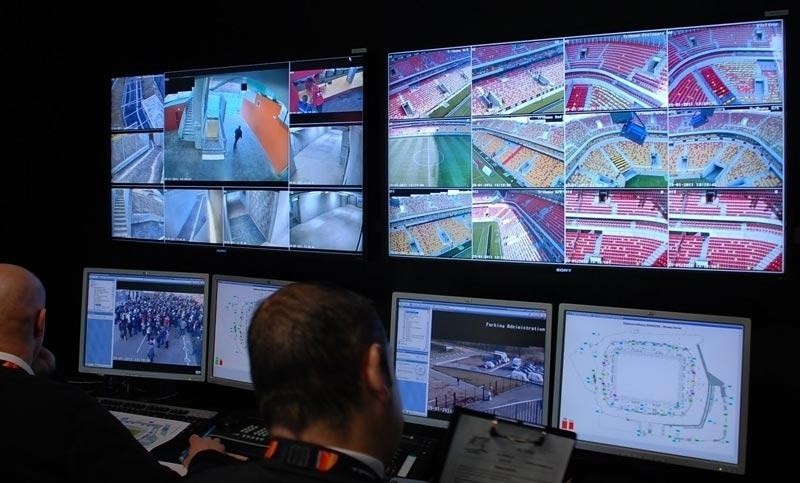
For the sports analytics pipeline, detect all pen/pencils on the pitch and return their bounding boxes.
[178,425,216,461]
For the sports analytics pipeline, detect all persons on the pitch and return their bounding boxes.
[0,263,183,483]
[181,283,404,483]
[233,126,242,146]
[114,294,204,362]
[298,73,324,113]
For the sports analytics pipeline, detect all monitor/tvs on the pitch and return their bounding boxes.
[388,290,554,433]
[76,267,210,383]
[93,41,377,264]
[373,6,797,282]
[549,301,752,477]
[206,274,299,392]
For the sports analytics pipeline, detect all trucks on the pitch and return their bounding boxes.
[483,350,508,368]
[512,351,543,383]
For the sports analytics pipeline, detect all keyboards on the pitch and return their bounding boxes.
[89,394,220,448]
[210,415,441,479]
